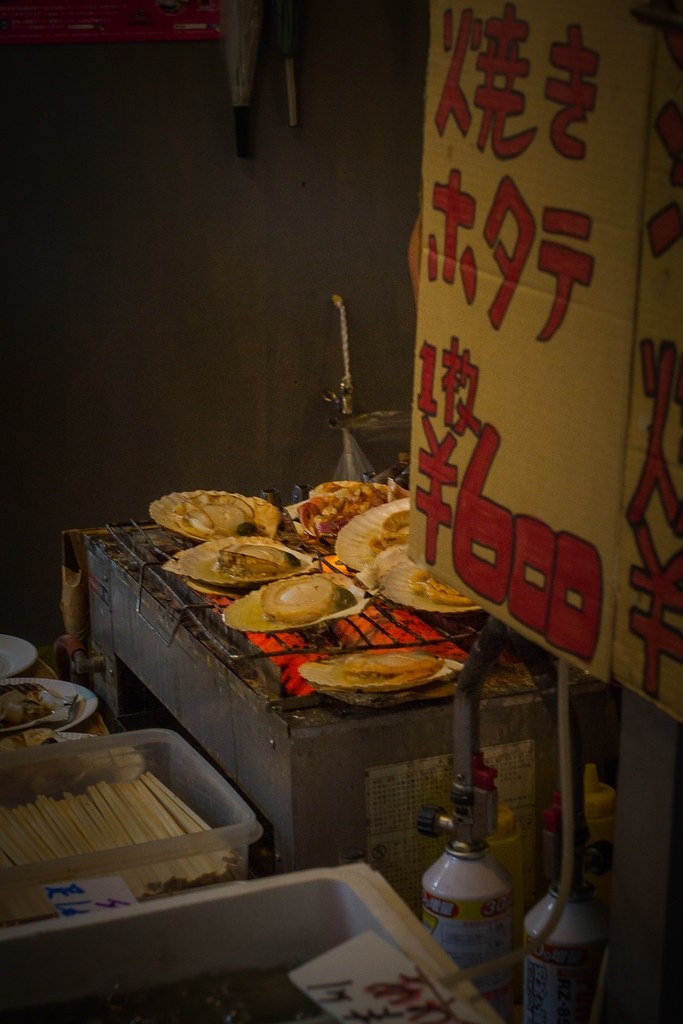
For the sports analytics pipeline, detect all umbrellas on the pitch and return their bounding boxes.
[213,0,274,161]
[271,0,308,129]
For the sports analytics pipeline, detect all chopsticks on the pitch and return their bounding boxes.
[0,771,248,930]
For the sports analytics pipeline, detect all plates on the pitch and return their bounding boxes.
[0,634,39,678]
[57,731,98,743]
[0,678,98,732]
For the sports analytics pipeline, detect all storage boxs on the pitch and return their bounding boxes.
[1,863,503,1023]
[0,727,263,929]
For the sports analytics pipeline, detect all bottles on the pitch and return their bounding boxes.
[540,763,618,887]
